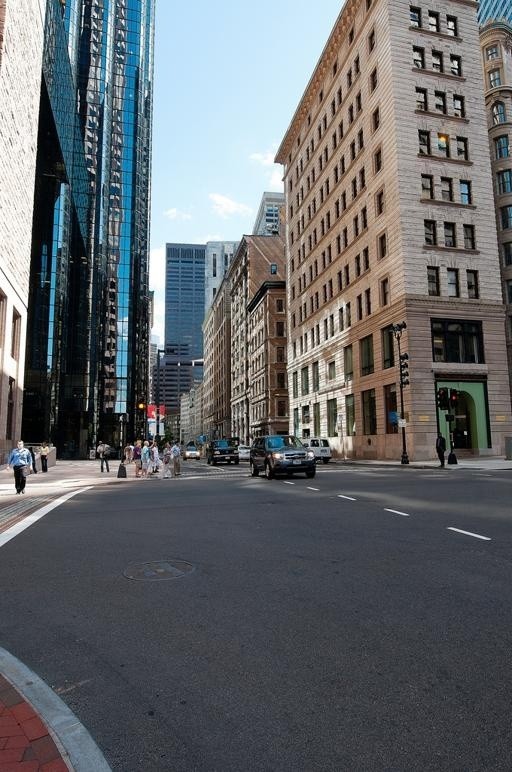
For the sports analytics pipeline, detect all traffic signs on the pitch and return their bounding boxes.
[398,417,407,428]
[388,411,398,425]
[404,412,409,423]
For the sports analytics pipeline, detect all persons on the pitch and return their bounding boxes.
[97,441,111,472]
[39,442,49,472]
[124,440,181,479]
[6,440,33,495]
[436,433,446,468]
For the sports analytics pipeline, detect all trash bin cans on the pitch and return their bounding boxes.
[505,437,512,460]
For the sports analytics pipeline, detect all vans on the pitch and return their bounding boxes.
[300,437,334,465]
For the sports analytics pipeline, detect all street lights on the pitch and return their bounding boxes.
[157,347,169,439]
[389,321,412,465]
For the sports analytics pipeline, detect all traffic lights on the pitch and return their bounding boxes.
[450,388,459,408]
[113,412,125,424]
[435,387,449,411]
[445,413,455,421]
[137,397,144,409]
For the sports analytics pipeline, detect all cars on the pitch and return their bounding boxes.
[237,444,252,461]
[177,444,209,457]
[183,447,201,461]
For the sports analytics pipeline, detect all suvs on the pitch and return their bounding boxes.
[207,439,242,467]
[250,434,318,481]
